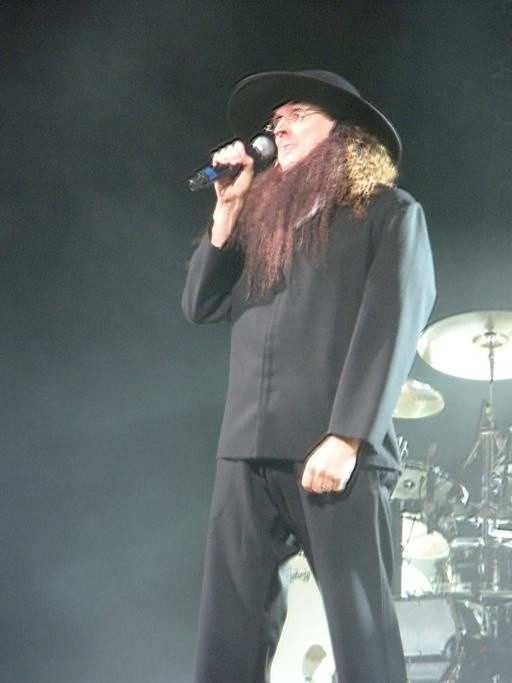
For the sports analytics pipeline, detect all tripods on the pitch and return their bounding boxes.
[395,343,512,683]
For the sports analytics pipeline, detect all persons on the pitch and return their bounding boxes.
[182,69,438,682]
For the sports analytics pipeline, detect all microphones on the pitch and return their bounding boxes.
[187,136,275,192]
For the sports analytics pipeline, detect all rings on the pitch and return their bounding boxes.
[323,485,332,490]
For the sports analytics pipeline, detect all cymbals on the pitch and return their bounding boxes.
[416,309,512,380]
[401,517,449,559]
[391,378,444,419]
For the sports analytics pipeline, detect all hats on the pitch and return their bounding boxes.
[225,70,402,165]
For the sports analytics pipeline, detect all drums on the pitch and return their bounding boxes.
[270,544,465,683]
[386,461,469,510]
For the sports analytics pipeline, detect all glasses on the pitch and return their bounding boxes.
[264,109,316,132]
